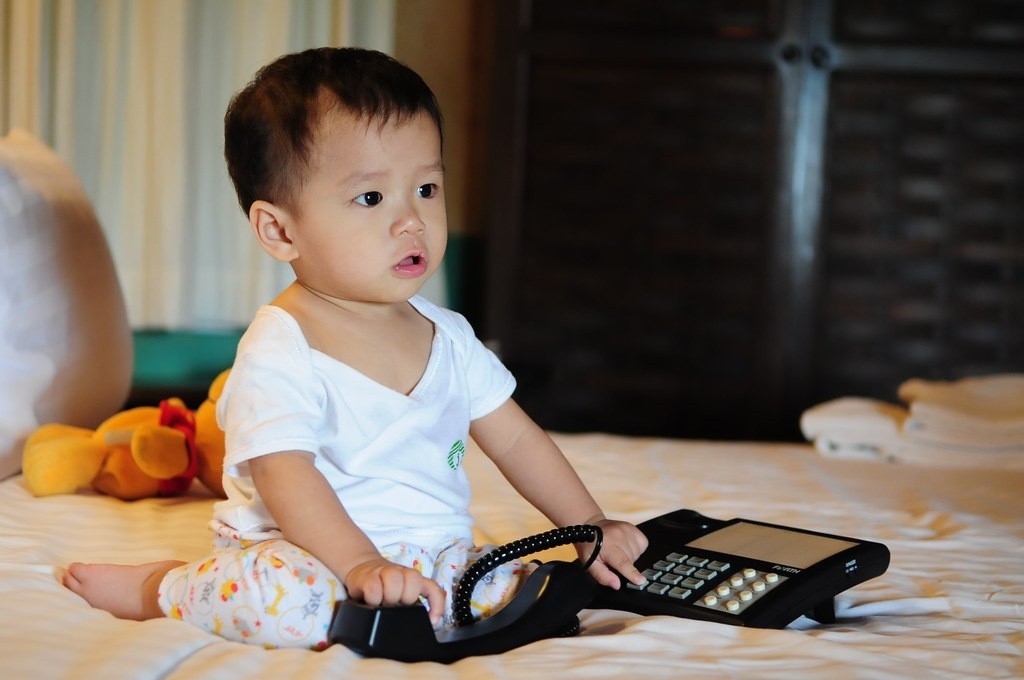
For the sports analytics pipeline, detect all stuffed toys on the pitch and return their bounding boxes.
[20,367,230,500]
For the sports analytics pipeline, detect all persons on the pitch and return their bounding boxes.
[62,44,648,651]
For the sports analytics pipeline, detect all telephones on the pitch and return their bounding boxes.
[326,508,890,664]
